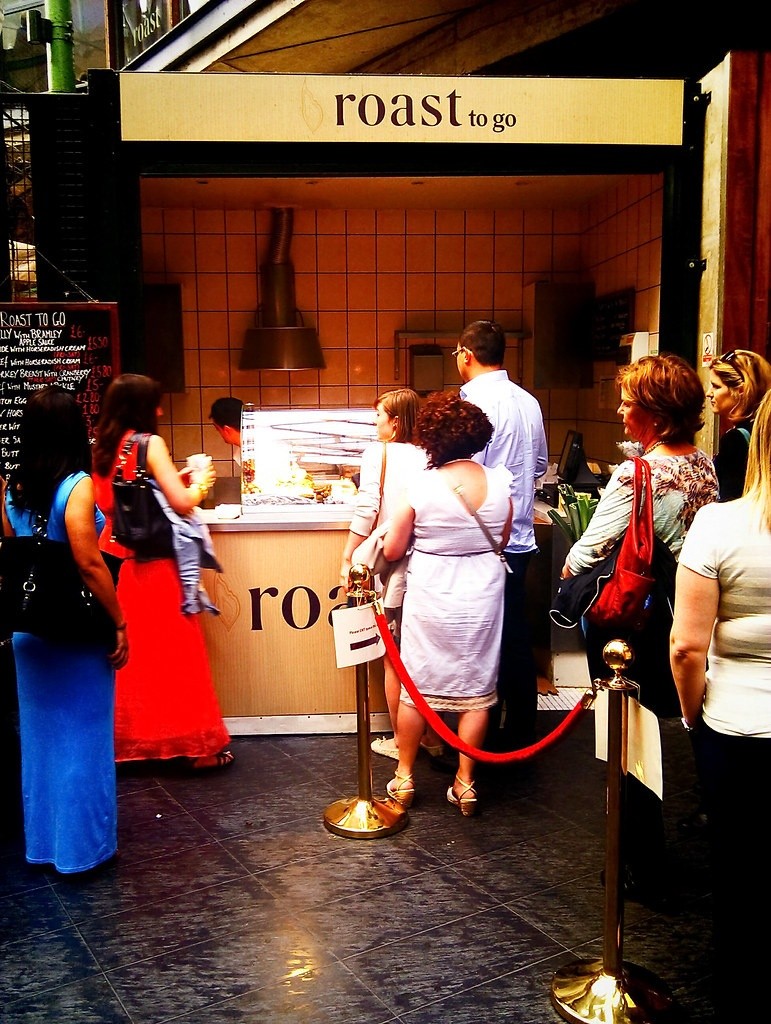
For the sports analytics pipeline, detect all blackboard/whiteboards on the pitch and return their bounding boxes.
[0,302,121,486]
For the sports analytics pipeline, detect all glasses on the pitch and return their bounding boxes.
[721,351,745,383]
[451,346,472,358]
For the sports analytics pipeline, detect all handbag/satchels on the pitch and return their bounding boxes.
[109,431,177,564]
[0,476,124,656]
[588,457,654,624]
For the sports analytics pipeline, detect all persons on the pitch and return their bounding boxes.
[457,320,549,750]
[93,374,235,772]
[0,387,128,873]
[705,350,771,503]
[562,355,721,902]
[210,398,251,466]
[382,395,513,817]
[339,389,444,758]
[669,388,771,1024]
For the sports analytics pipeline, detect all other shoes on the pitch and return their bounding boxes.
[500,748,538,789]
[475,740,504,782]
[72,849,121,886]
[420,736,444,757]
[370,736,400,761]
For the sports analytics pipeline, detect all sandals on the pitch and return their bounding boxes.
[116,761,160,778]
[171,750,236,778]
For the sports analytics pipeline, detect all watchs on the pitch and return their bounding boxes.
[191,483,208,500]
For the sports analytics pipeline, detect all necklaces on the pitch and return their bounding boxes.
[444,458,470,465]
[643,440,668,455]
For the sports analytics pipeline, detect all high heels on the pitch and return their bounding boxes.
[444,774,478,817]
[385,769,415,808]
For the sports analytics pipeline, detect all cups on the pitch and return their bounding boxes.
[185,454,212,484]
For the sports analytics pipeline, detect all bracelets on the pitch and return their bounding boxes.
[118,623,127,630]
[681,717,694,732]
